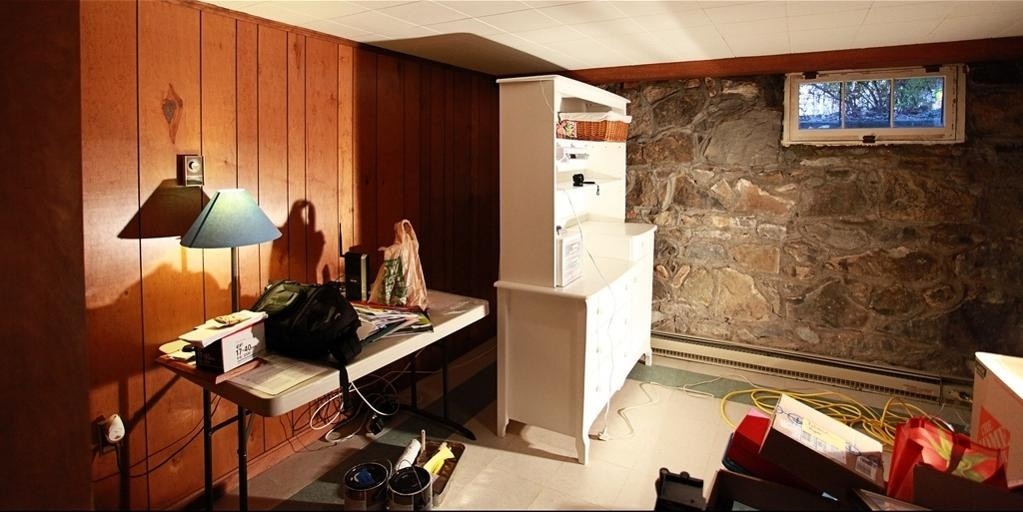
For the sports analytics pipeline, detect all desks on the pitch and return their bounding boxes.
[155,286,489,512]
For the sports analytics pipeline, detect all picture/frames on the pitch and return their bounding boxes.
[846,485,930,511]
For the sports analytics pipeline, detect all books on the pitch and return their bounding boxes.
[347,300,433,346]
[177,308,269,350]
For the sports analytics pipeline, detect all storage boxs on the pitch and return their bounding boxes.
[758,393,885,495]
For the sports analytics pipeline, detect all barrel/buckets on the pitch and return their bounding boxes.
[388,460,431,511]
[343,459,393,511]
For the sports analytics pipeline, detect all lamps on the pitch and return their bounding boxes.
[181,189,284,311]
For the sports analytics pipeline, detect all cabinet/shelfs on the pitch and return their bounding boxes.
[971,351,1023,482]
[493,74,657,465]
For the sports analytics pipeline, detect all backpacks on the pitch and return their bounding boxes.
[251,279,363,367]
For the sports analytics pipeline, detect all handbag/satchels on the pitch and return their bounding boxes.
[886,414,1012,503]
[366,217,430,313]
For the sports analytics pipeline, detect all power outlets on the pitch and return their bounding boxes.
[98,421,126,456]
